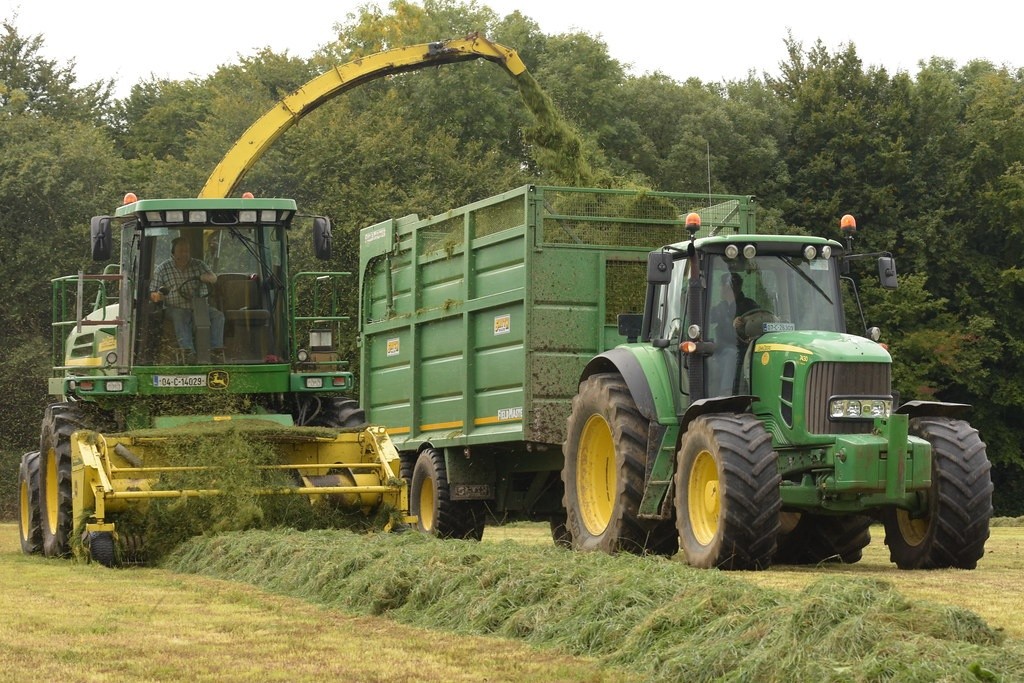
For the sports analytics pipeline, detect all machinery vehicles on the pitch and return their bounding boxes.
[18,33,533,571]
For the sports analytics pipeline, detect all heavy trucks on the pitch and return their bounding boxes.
[354,182,995,571]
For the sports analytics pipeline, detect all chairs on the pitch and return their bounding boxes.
[216,274,271,325]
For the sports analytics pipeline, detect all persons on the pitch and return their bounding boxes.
[710,275,763,350]
[150,238,227,365]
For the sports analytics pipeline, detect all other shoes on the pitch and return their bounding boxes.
[178,350,198,366]
[210,351,224,364]
